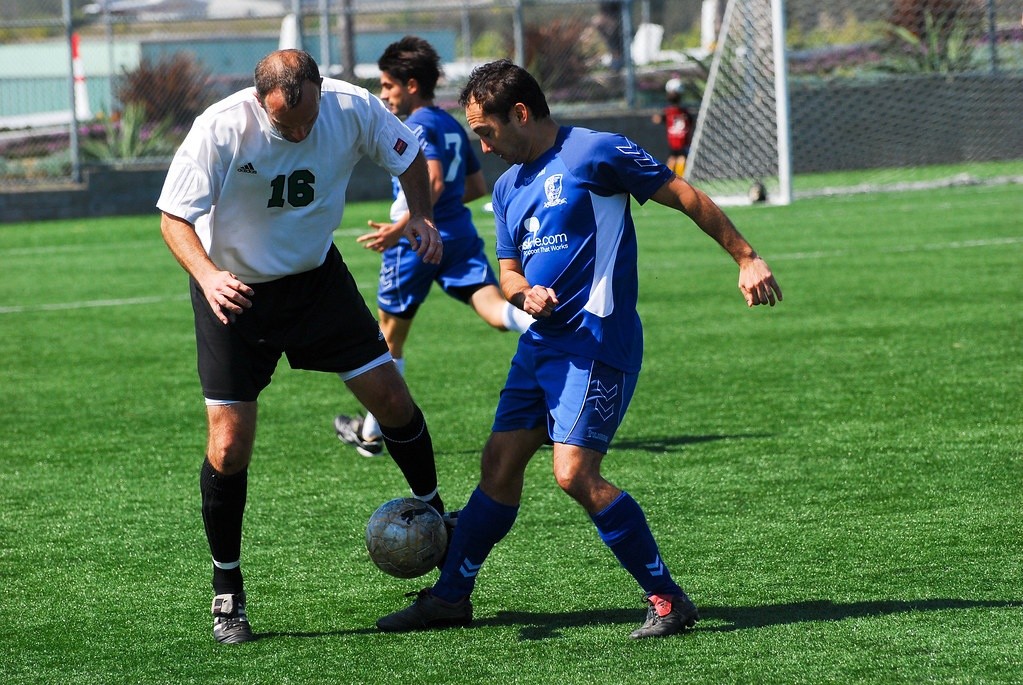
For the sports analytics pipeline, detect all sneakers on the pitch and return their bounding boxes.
[628,585,700,639]
[436,510,458,572]
[376,587,474,632]
[335,412,385,458]
[211,590,252,645]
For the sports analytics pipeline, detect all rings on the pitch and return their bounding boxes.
[437,241,442,244]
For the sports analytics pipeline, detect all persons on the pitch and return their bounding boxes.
[653,92,696,177]
[336,35,537,459]
[375,59,783,639]
[156,49,444,645]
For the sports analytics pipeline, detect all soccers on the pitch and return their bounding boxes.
[363,496,449,579]
